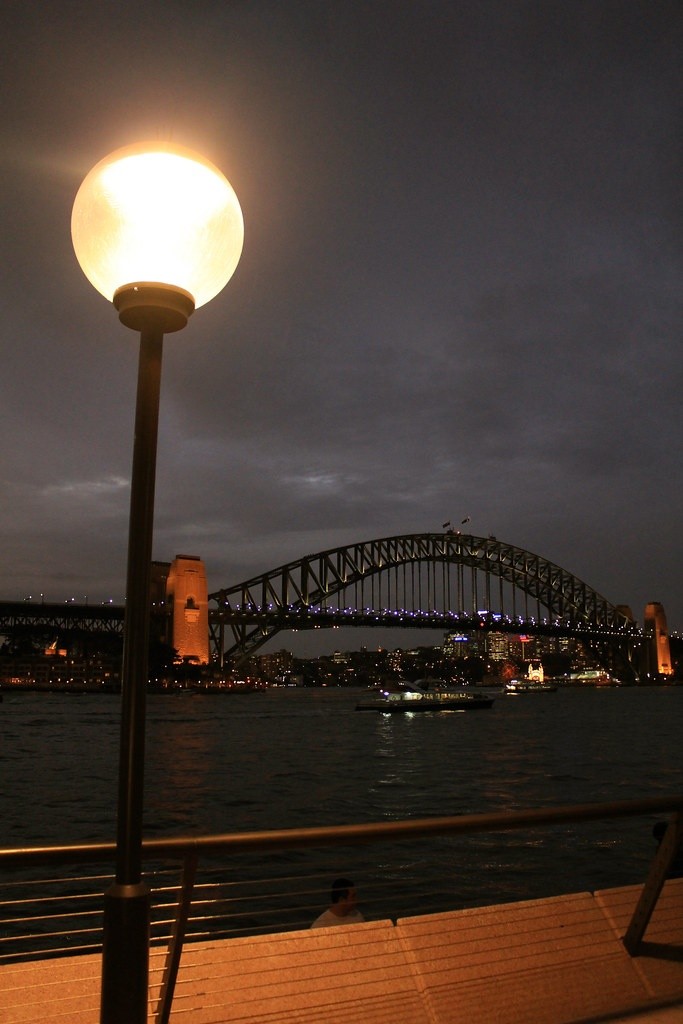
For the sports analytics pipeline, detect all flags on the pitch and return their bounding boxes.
[462,519,468,524]
[443,521,450,528]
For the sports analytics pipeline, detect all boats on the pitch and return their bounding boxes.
[355,692,502,714]
[503,677,558,696]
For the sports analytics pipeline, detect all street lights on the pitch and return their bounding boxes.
[70,140,245,1024]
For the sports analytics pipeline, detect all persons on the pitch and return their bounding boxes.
[310,878,364,928]
[652,821,683,880]
